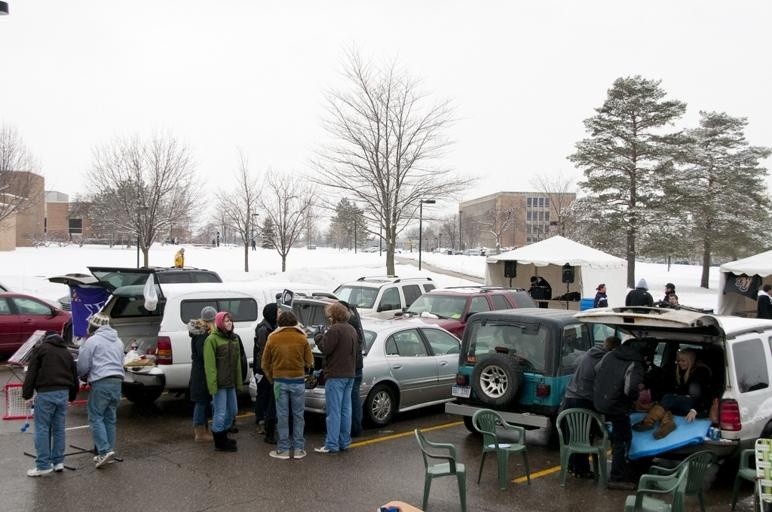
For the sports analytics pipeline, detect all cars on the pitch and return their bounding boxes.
[248,289,466,431]
[1,284,78,360]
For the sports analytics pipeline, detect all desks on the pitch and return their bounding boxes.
[536,299,581,309]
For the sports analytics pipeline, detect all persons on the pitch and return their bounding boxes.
[313,301,359,453]
[203,311,244,449]
[263,386,278,445]
[340,301,364,438]
[625,278,654,313]
[20,329,80,477]
[227,334,247,433]
[253,303,283,435]
[188,305,218,443]
[260,311,314,460]
[251,239,257,251]
[756,284,772,319]
[594,284,608,307]
[563,336,621,479]
[174,248,185,268]
[76,312,126,469]
[659,283,675,308]
[592,336,660,490]
[631,346,714,439]
[528,275,552,308]
[665,294,680,310]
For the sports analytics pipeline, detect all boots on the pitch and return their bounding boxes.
[194,424,215,442]
[653,410,676,439]
[206,429,213,436]
[227,437,237,445]
[213,430,237,451]
[632,405,666,431]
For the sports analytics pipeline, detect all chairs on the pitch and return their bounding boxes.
[414,428,467,511]
[556,408,609,486]
[732,449,759,512]
[650,450,717,512]
[625,462,689,512]
[472,409,530,490]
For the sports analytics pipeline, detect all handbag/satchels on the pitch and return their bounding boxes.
[304,375,317,388]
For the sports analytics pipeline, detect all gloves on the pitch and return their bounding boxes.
[314,326,321,343]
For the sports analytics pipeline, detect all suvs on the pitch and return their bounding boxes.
[445,308,584,446]
[394,287,537,340]
[332,276,438,320]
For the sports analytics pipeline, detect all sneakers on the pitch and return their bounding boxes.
[269,449,290,459]
[264,436,277,444]
[94,454,114,463]
[230,426,238,433]
[96,451,114,467]
[314,446,331,452]
[294,449,307,458]
[27,468,53,476]
[567,465,574,473]
[608,481,637,489]
[257,426,266,433]
[51,462,64,470]
[575,472,594,480]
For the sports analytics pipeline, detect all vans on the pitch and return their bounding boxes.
[574,306,772,466]
[55,266,223,311]
[84,266,268,405]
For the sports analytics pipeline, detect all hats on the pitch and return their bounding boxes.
[201,306,217,321]
[636,278,647,288]
[90,313,110,328]
[531,277,537,282]
[666,283,675,289]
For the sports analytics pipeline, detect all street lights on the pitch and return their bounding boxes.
[250,213,261,251]
[419,199,436,271]
